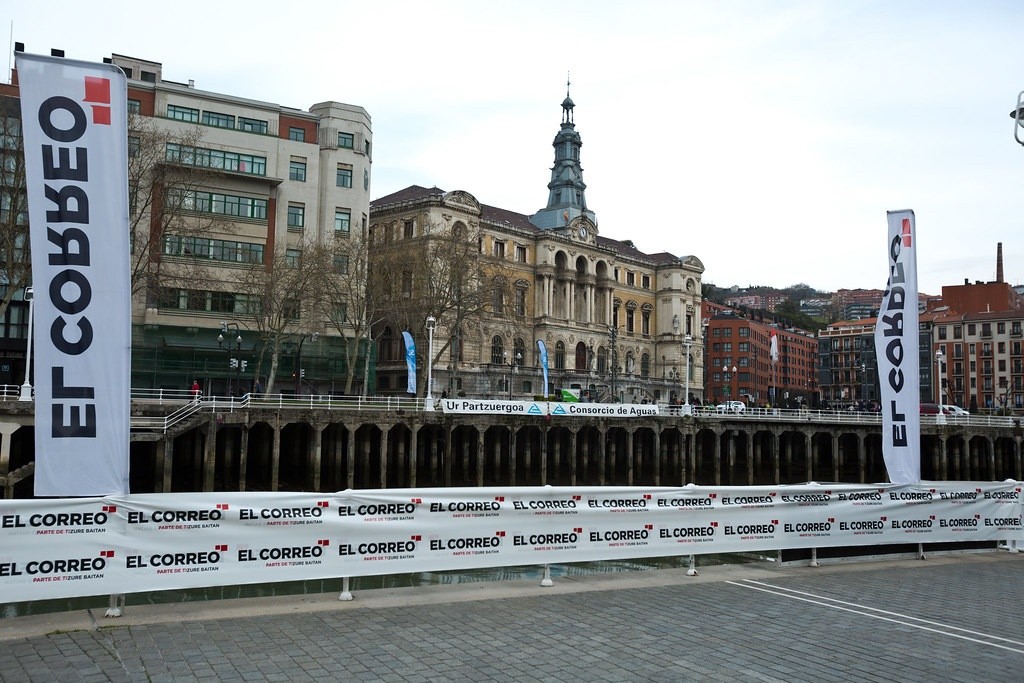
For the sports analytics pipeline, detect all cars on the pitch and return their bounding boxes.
[715,400,746,415]
[942,404,971,416]
[920,403,952,416]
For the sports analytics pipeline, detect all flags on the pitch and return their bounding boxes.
[770,327,779,363]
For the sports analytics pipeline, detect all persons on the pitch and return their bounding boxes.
[641,396,647,404]
[441,389,447,398]
[848,400,882,416]
[801,398,809,416]
[631,396,639,404]
[791,397,800,416]
[191,380,199,400]
[733,398,778,415]
[582,392,590,403]
[668,393,723,415]
[459,388,466,397]
[969,399,978,415]
[614,395,621,404]
[253,380,263,402]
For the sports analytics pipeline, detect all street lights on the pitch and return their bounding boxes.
[425,317,436,412]
[500,352,522,401]
[216,333,243,394]
[682,334,693,414]
[600,322,627,402]
[723,365,738,411]
